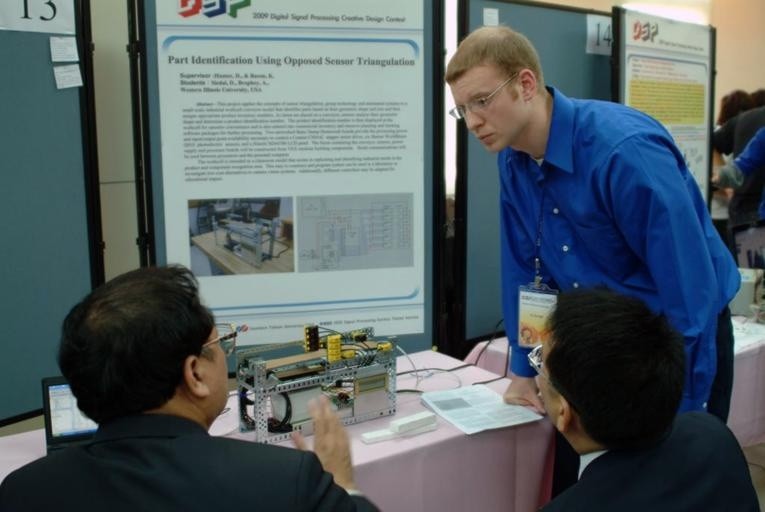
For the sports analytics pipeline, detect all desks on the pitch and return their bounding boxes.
[478,309,765,450]
[1,350,556,512]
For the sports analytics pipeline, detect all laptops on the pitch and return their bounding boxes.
[42,375,101,457]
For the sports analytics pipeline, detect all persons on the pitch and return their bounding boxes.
[447,27,742,497]
[712,90,765,268]
[0,263,382,512]
[527,285,760,511]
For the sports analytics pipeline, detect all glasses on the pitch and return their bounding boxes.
[202,322,238,358]
[449,73,519,120]
[527,344,548,382]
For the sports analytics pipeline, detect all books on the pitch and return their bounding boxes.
[419,383,544,436]
